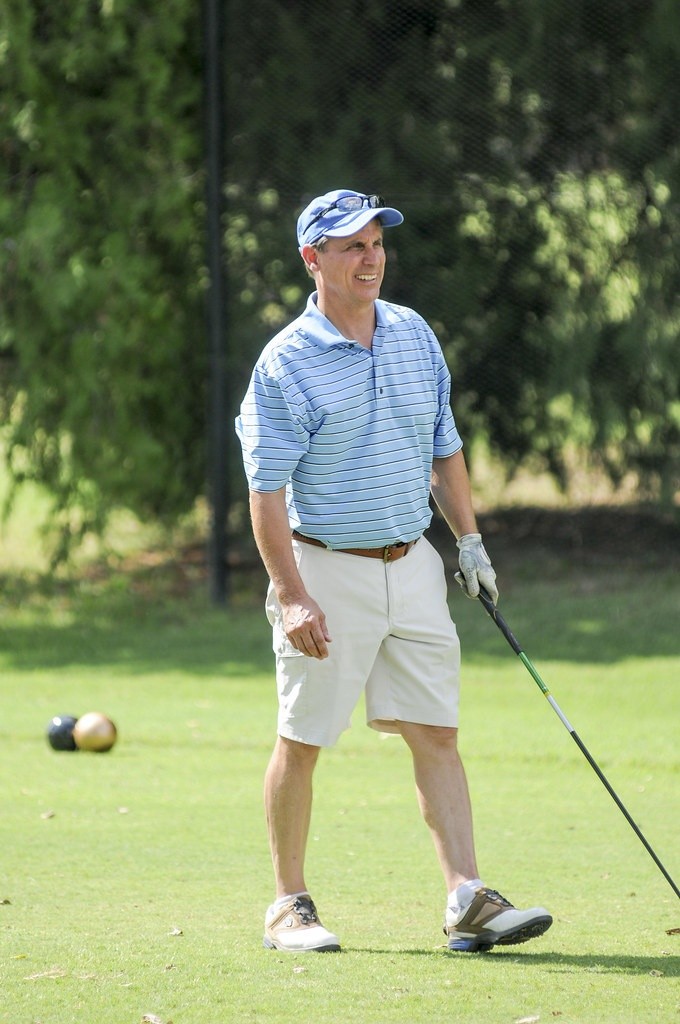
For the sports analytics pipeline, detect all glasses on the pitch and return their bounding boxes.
[303,193,386,236]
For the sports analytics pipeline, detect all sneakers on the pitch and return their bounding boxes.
[261,894,341,954]
[443,887,553,952]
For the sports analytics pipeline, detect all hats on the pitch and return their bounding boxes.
[297,189,404,252]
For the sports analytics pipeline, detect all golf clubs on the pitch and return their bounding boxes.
[457,565,680,901]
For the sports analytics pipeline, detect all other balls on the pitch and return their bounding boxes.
[48,711,117,753]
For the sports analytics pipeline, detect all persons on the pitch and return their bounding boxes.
[235,189,554,954]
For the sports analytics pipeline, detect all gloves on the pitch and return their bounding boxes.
[454,532,498,608]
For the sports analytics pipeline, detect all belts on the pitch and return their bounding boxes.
[291,530,420,564]
